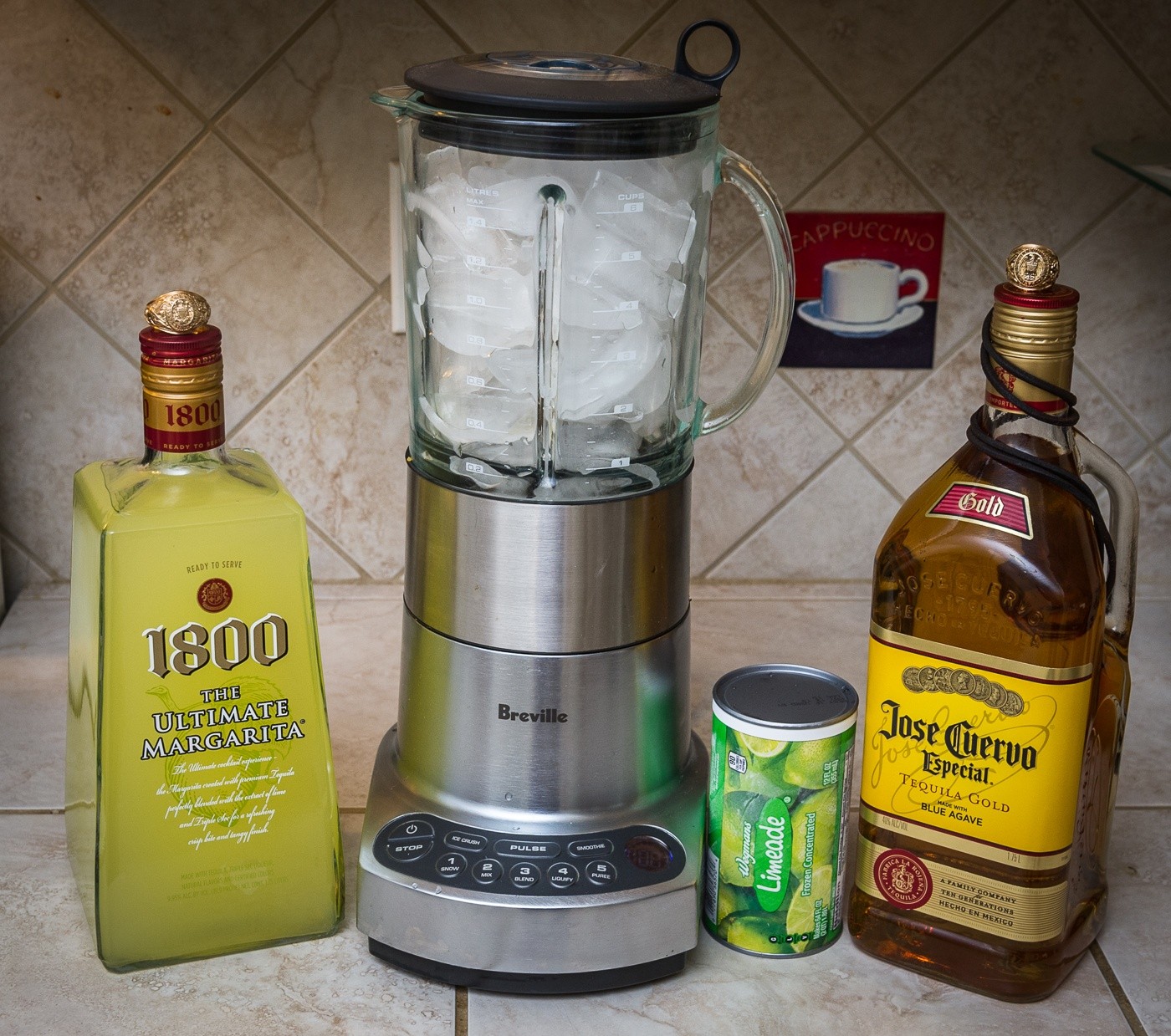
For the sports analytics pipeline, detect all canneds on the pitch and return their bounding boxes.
[702,664,859,959]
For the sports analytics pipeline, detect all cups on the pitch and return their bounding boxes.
[819,259,927,325]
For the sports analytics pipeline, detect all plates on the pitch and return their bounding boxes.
[798,300,923,339]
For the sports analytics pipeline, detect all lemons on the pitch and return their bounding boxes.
[718,733,840,951]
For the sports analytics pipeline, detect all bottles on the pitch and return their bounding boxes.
[848,244,1137,1003]
[66,290,344,970]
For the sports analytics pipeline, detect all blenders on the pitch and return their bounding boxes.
[356,20,794,1000]
